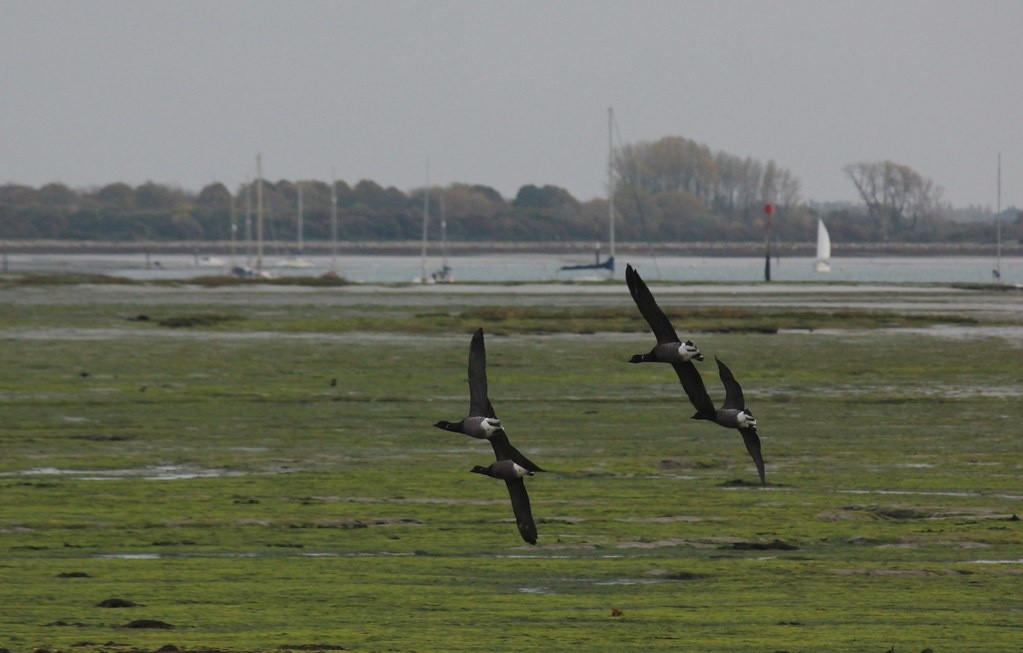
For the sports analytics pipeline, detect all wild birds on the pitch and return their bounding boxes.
[432,263,765,546]
